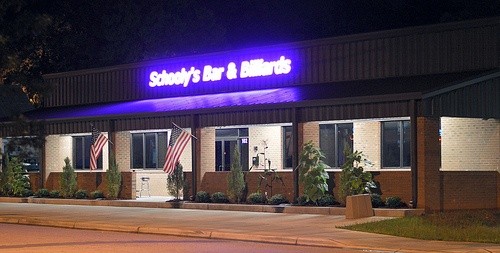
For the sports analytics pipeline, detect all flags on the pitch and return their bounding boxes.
[161,126,191,176]
[88,127,107,172]
